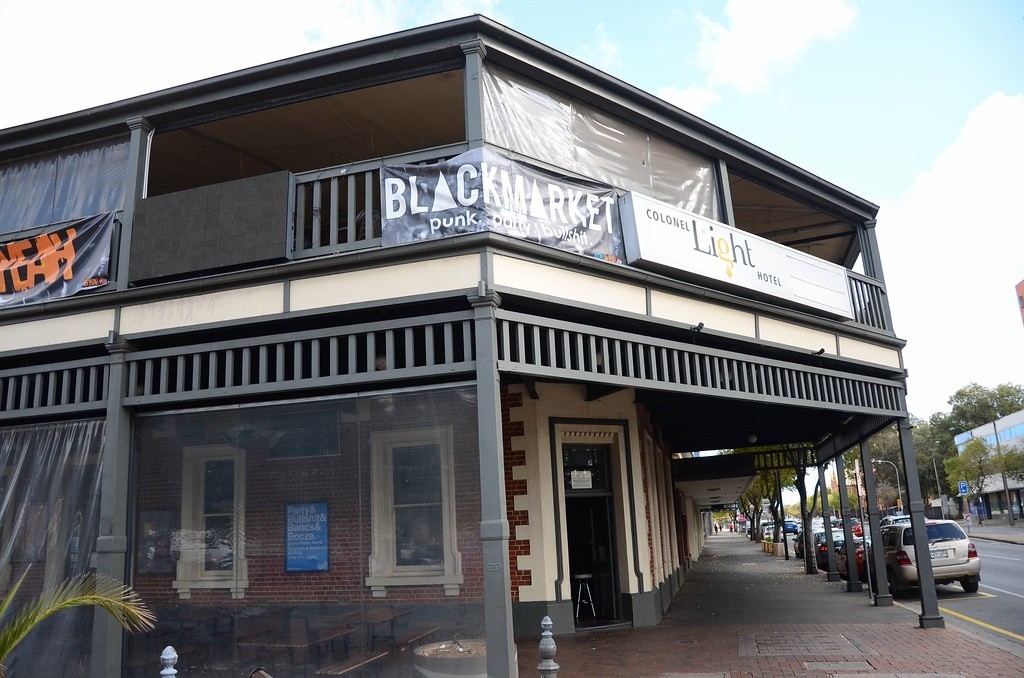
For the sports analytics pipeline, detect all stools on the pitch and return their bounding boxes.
[574,574,596,618]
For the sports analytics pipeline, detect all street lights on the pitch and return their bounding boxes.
[872,460,904,516]
[932,440,946,520]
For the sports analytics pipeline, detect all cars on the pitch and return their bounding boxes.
[744,512,929,582]
[861,518,981,599]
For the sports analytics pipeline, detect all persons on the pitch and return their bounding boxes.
[714,522,733,535]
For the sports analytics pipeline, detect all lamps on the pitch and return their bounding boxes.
[107,330,116,344]
[691,322,704,332]
[477,280,487,297]
[812,349,826,356]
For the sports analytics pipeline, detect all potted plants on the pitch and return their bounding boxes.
[761,536,784,556]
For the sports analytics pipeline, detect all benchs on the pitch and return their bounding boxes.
[126,624,442,678]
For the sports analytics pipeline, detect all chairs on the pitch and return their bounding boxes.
[356,209,382,241]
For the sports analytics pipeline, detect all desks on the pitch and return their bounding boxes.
[145,606,414,678]
